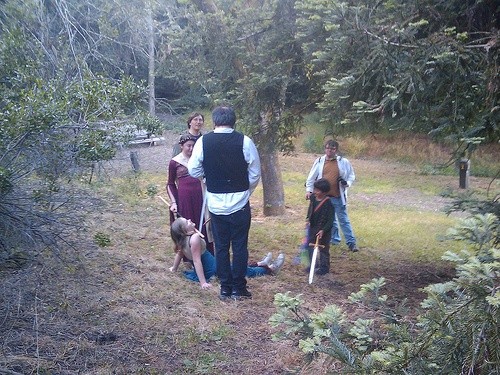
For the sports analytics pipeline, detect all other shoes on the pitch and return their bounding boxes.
[316,268,329,275]
[304,264,318,272]
[220,286,252,301]
[348,243,358,252]
[261,252,285,275]
[332,239,340,245]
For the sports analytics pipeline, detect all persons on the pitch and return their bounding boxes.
[166,106,360,300]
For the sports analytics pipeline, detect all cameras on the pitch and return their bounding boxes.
[338,176,347,185]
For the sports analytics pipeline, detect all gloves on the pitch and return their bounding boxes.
[337,175,347,184]
